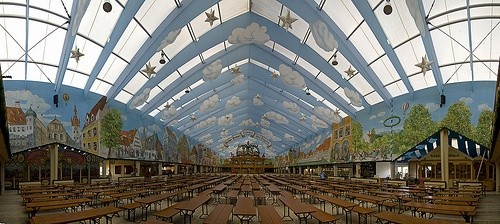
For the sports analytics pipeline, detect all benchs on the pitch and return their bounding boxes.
[19,173,487,224]
